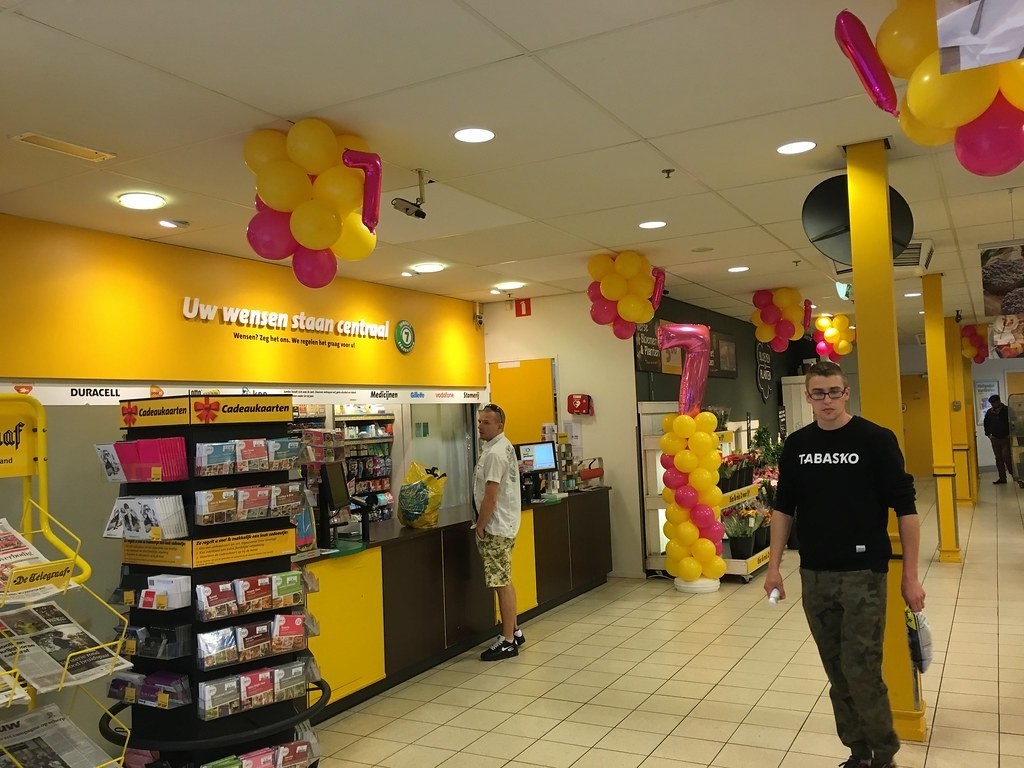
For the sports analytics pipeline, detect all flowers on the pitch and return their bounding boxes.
[715,448,779,485]
[718,505,774,536]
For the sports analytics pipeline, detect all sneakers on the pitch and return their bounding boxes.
[480,628,525,661]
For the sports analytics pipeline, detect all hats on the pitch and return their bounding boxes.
[988,395,1000,401]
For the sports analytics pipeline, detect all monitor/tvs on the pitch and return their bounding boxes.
[517,440,558,500]
[319,460,350,549]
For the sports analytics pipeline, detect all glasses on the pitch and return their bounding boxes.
[484,404,502,422]
[807,387,846,400]
[990,401,996,403]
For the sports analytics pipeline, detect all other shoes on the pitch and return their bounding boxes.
[993,479,1007,484]
[839,755,895,768]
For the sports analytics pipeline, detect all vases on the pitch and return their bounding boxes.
[719,467,753,492]
[729,526,771,559]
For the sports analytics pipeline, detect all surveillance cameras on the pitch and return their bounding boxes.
[477,315,484,326]
[393,199,426,219]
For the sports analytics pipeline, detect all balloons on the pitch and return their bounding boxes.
[586,251,665,339]
[241,118,382,288]
[834,0,1024,176]
[751,288,854,363]
[656,324,727,582]
[961,325,988,363]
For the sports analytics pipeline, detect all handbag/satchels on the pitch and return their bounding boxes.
[397,461,448,530]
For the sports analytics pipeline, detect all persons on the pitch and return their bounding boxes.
[100,449,157,533]
[983,395,1016,484]
[763,360,925,768]
[470,404,526,661]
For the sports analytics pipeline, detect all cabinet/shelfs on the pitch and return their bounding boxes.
[99,394,332,768]
[638,401,788,585]
[0,393,131,768]
[335,404,395,523]
[781,376,814,436]
